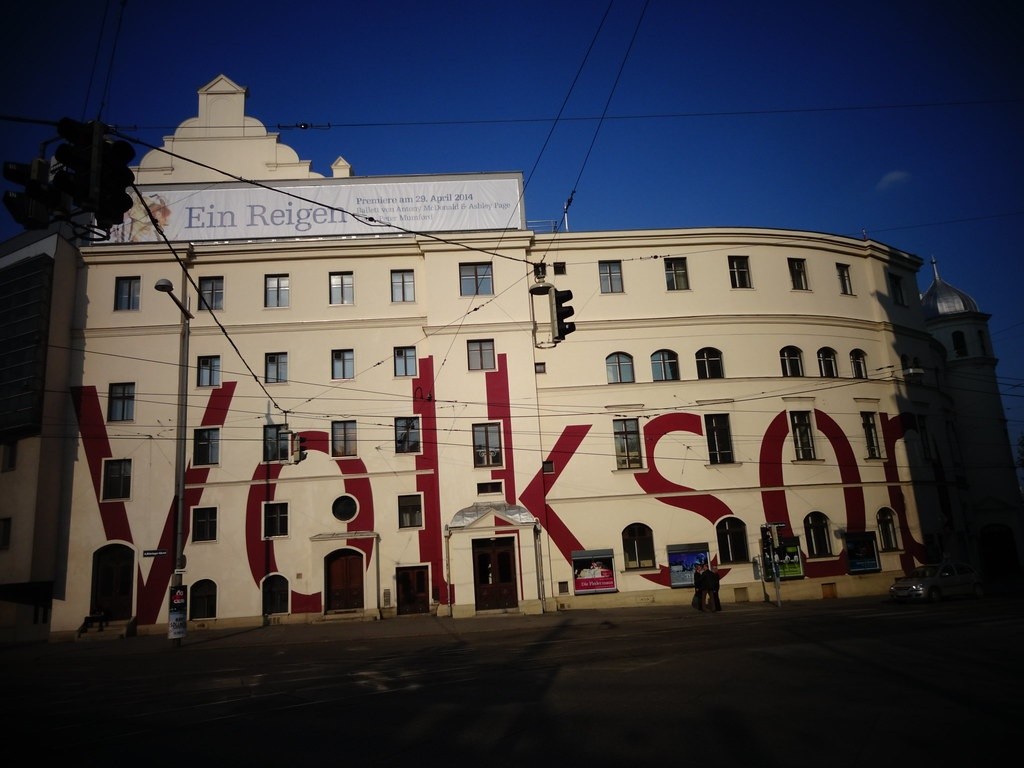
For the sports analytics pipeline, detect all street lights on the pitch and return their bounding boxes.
[154,278,195,651]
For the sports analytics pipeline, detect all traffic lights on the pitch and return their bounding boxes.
[771,524,784,549]
[761,527,771,548]
[548,287,576,343]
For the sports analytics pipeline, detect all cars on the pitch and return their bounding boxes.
[889,561,986,606]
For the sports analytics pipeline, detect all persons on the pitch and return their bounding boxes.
[692,563,722,612]
